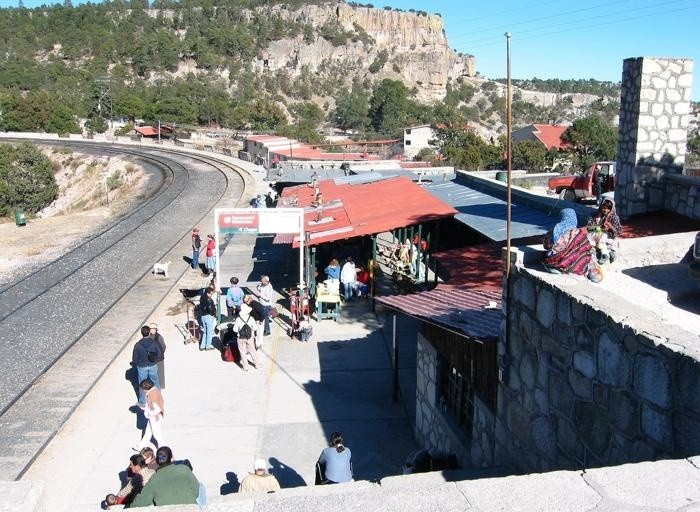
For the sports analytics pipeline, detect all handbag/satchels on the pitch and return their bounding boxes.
[224,342,242,362]
[147,350,158,363]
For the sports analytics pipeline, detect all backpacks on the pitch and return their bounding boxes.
[239,316,252,339]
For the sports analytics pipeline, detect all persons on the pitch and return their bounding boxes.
[252,191,281,208]
[205,234,216,272]
[542,163,624,283]
[105,445,204,510]
[324,254,369,302]
[199,273,273,371]
[132,326,161,406]
[404,232,428,261]
[149,323,166,389]
[237,459,280,493]
[131,379,164,452]
[316,431,352,486]
[192,227,200,269]
[293,316,312,342]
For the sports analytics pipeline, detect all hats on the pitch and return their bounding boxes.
[238,303,253,316]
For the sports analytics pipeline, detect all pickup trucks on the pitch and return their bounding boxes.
[547,159,618,203]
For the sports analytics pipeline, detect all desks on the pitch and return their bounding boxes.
[317,279,341,322]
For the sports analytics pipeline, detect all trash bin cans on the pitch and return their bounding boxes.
[15,211,26,227]
[496,172,509,183]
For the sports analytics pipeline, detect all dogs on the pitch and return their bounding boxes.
[152,259,172,278]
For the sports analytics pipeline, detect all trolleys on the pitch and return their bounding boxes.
[287,287,310,340]
